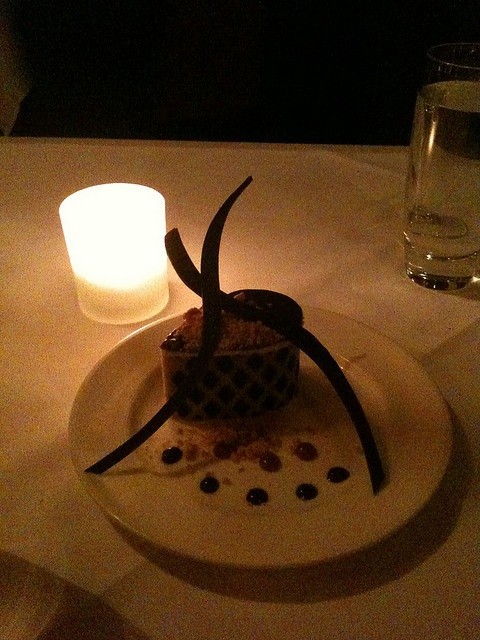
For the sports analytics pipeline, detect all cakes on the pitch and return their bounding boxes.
[85,174,386,496]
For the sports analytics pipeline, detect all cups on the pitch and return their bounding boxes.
[404,42,480,291]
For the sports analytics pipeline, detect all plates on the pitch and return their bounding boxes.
[69,305,454,569]
